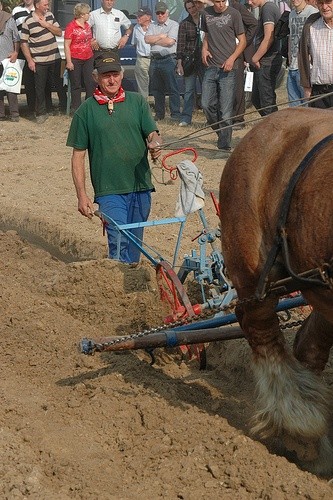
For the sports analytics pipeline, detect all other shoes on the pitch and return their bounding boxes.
[37,113,47,124]
[171,117,179,121]
[213,150,228,158]
[154,116,160,121]
[233,121,243,129]
[11,116,19,122]
[179,122,187,126]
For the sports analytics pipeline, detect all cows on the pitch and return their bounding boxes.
[214,103,333,468]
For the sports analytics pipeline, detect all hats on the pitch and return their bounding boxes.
[154,2,168,13]
[134,7,151,16]
[94,52,122,74]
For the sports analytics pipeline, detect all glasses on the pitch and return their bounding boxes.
[315,0,331,6]
[156,12,165,15]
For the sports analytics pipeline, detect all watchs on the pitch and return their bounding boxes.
[125,33,130,37]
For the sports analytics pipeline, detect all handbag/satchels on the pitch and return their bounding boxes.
[0,59,26,94]
[244,66,254,91]
[183,57,197,76]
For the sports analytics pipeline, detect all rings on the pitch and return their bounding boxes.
[178,73,180,76]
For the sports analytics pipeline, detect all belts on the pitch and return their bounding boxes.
[145,57,151,59]
[153,53,174,59]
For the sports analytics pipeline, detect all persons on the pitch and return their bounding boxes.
[66,53,163,264]
[86,0,133,55]
[300,0,333,109]
[200,0,247,150]
[0,0,20,122]
[12,0,53,111]
[64,3,97,117]
[131,0,320,130]
[20,0,67,124]
